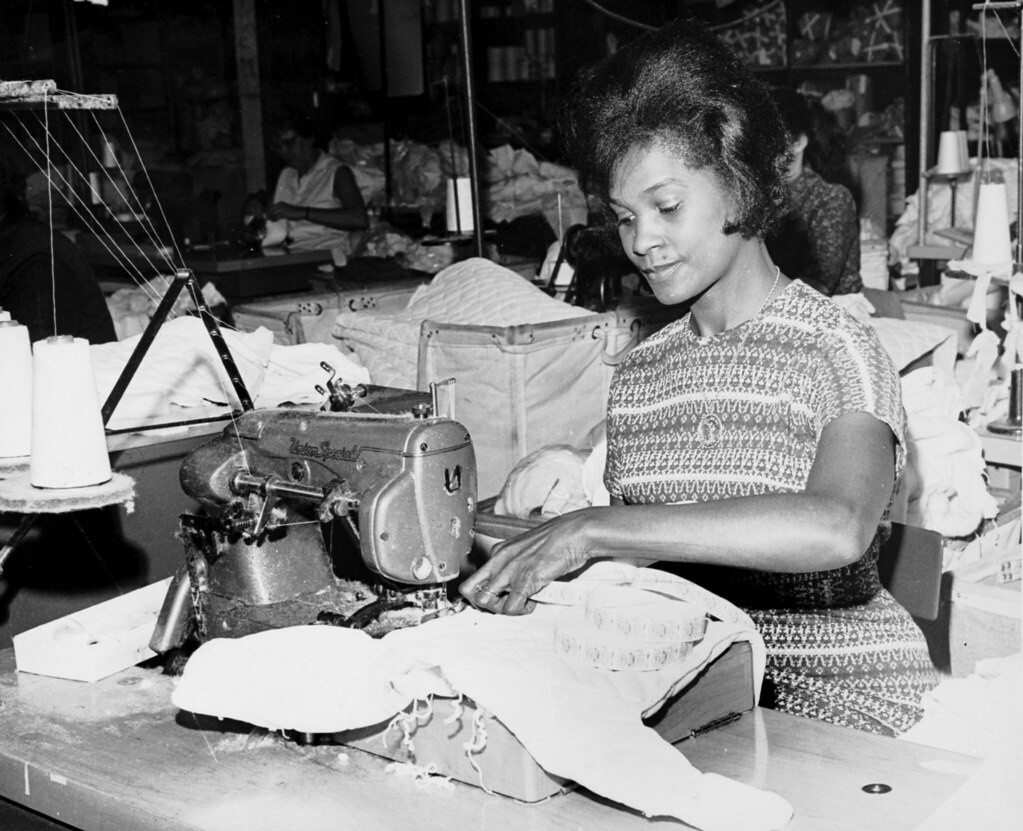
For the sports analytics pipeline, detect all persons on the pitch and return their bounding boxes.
[762,92,865,297]
[459,46,935,738]
[265,111,369,274]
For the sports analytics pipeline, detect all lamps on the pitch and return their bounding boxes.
[987,69,1016,122]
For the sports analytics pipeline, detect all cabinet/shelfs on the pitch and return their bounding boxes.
[422,0,919,234]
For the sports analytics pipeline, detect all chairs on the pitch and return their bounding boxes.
[879,521,944,621]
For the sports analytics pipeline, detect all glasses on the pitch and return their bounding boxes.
[278,132,300,147]
